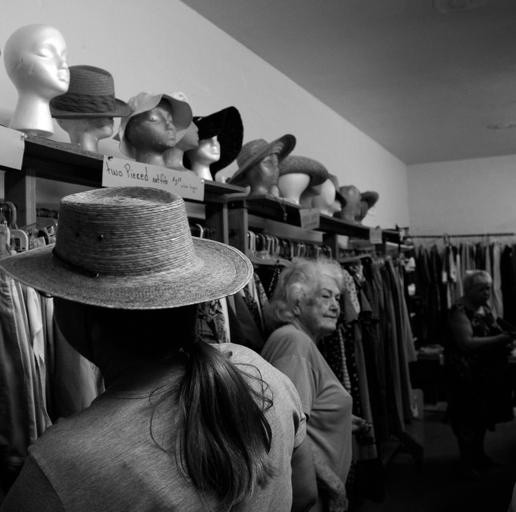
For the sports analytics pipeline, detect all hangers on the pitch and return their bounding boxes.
[247,230,391,277]
[0,200,59,272]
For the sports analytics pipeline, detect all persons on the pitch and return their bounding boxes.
[163,92,199,172]
[116,92,194,167]
[324,191,346,217]
[2,23,73,136]
[49,63,131,153]
[260,254,355,511]
[439,268,516,466]
[339,185,380,222]
[229,134,298,194]
[311,169,339,211]
[278,155,328,203]
[0,183,321,512]
[181,105,243,185]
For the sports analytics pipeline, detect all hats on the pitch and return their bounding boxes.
[118,90,194,160]
[362,190,377,205]
[0,187,254,310]
[278,155,329,200]
[339,184,361,205]
[181,106,245,177]
[50,66,134,118]
[226,133,297,187]
[328,173,347,206]
